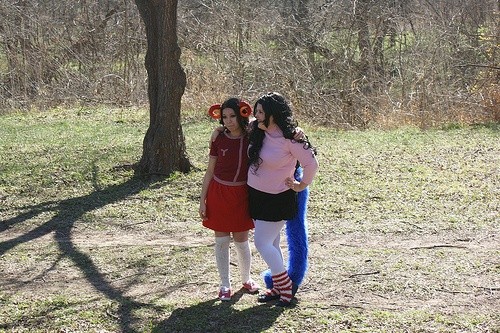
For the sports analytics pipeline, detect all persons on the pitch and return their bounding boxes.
[199,97,306,301]
[208,91,319,307]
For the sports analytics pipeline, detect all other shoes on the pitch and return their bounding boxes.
[275,283,298,306]
[218,288,231,300]
[242,279,259,292]
[258,293,280,302]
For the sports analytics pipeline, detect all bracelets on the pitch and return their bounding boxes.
[215,128,221,133]
